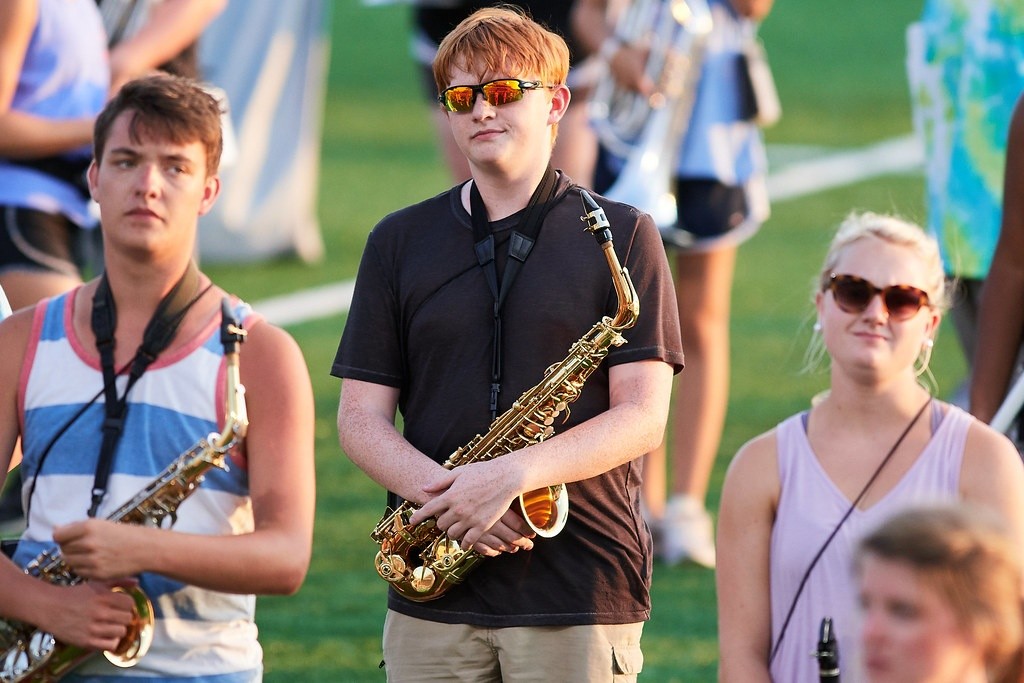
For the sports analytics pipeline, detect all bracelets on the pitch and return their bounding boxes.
[591,28,629,64]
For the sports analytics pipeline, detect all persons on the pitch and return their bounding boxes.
[839,495,1023,682]
[899,0,1021,419]
[974,94,1024,453]
[4,70,320,682]
[563,0,780,572]
[710,203,1024,683]
[410,0,602,223]
[1,1,178,314]
[92,0,228,155]
[326,2,686,672]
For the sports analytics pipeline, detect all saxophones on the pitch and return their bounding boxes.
[1,299,248,683]
[371,189,640,604]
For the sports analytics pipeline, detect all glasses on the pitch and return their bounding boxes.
[439,78,555,112]
[821,273,936,322]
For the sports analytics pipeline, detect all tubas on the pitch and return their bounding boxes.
[590,0,714,233]
